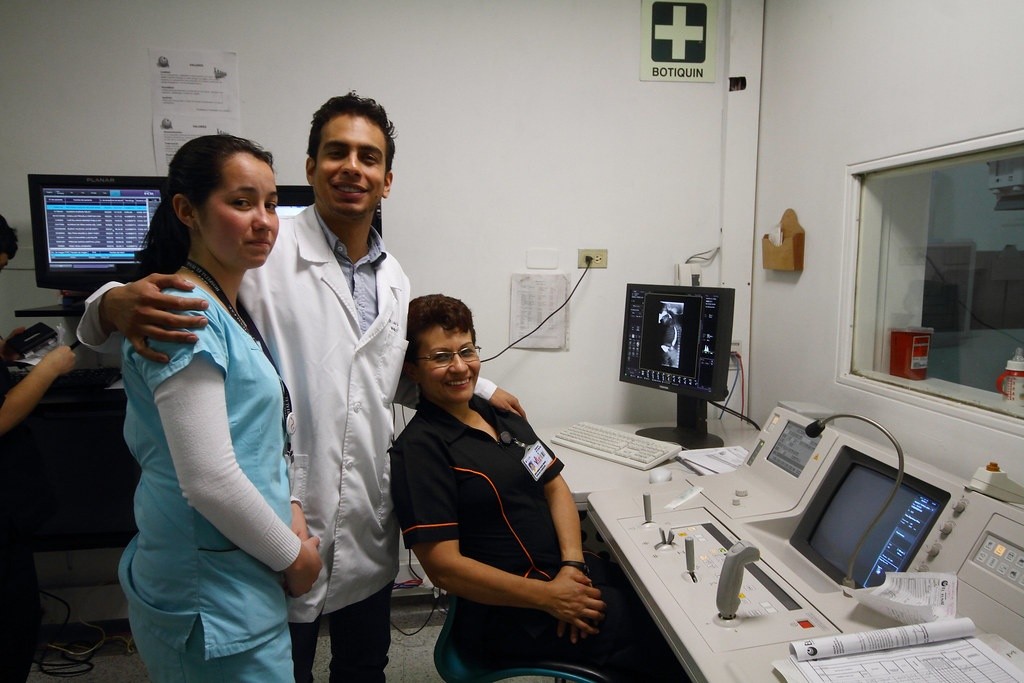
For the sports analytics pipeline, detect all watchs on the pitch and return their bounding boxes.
[560,561,592,577]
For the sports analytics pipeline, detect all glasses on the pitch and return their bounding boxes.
[416,345,481,368]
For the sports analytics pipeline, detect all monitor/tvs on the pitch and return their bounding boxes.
[26,174,170,290]
[619,283,734,400]
[809,464,945,588]
[274,185,383,237]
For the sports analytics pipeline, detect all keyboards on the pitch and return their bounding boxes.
[551,420,682,470]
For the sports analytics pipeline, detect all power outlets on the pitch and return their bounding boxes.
[577,248,608,268]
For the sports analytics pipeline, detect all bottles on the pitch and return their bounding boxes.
[996,347,1024,408]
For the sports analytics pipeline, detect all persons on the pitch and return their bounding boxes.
[391,294,691,682]
[77,131,324,683]
[0,213,77,683]
[234,91,528,683]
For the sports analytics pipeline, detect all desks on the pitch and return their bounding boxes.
[530,419,763,513]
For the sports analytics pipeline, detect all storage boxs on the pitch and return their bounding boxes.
[887,325,935,381]
